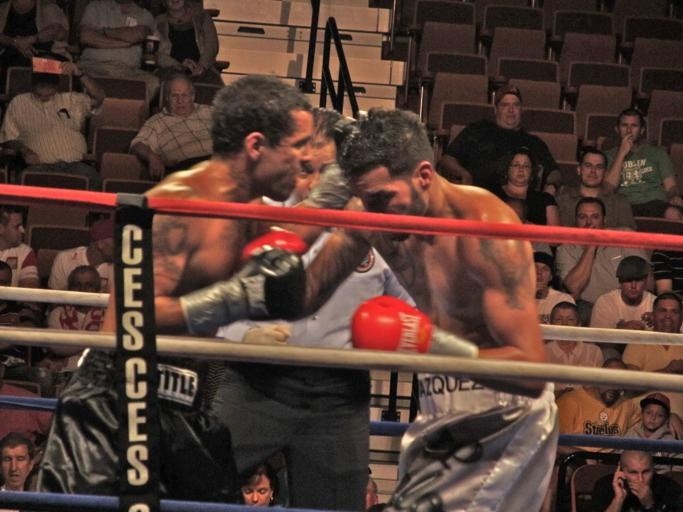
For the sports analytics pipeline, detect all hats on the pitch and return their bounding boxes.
[616,255,651,279]
[494,85,522,106]
[640,393,671,416]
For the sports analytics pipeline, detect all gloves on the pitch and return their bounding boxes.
[180,249,308,337]
[350,295,478,381]
[239,230,309,265]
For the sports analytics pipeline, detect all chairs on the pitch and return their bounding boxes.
[368,0,683,512]
[0,0,226,438]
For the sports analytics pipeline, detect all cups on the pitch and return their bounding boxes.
[143,35,160,65]
